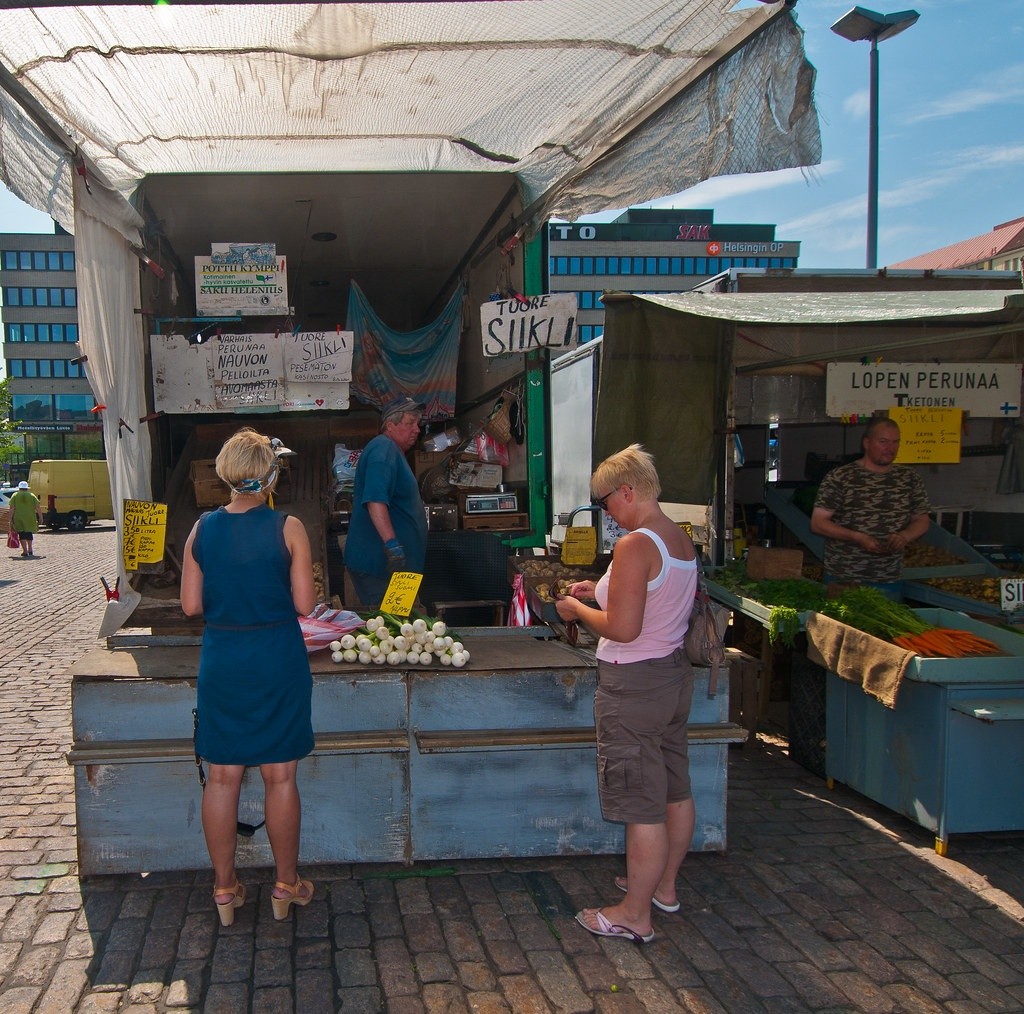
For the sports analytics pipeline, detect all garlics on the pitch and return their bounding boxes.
[330,606,470,667]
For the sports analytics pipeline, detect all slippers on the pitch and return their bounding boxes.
[574,906,654,943]
[614,874,679,913]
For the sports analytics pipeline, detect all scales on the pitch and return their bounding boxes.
[465,492,518,513]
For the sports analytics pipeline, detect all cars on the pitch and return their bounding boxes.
[1,487,19,509]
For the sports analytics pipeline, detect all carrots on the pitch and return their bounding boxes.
[814,585,1012,658]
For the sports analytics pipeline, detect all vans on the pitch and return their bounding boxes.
[27,459,116,531]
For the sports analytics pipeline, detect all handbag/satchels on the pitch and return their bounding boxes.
[6,529,19,549]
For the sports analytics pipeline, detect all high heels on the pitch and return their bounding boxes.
[213,881,246,927]
[270,874,314,920]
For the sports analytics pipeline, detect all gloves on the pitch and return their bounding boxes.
[382,535,407,570]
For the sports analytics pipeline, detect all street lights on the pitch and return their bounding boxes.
[829,5,920,273]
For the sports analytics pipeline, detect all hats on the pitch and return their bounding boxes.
[17,481,31,489]
[380,394,426,419]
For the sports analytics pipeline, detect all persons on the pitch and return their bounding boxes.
[7,481,43,556]
[179,430,318,927]
[554,442,703,945]
[343,396,429,608]
[810,418,932,602]
